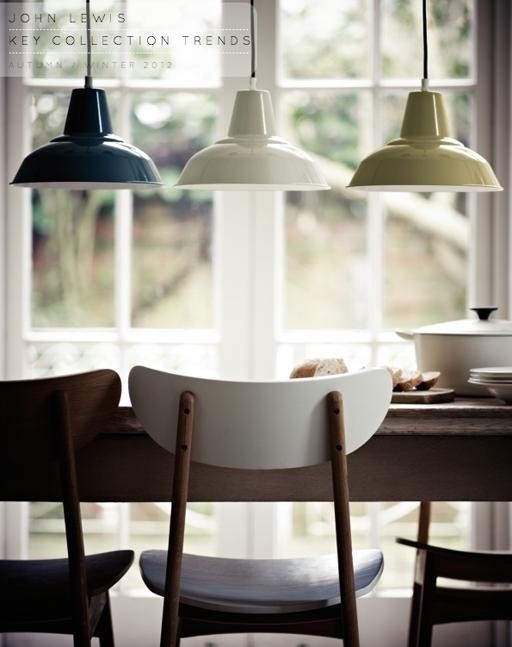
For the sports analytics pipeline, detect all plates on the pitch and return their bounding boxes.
[393,388,455,403]
[466,366,512,399]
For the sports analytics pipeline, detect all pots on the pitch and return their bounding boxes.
[395,305,512,399]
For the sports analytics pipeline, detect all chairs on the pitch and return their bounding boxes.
[397,501,512,646]
[0,370,134,646]
[129,365,394,646]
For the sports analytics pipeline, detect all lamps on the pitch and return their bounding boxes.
[346,0,504,192]
[173,0,331,191]
[9,0,163,189]
